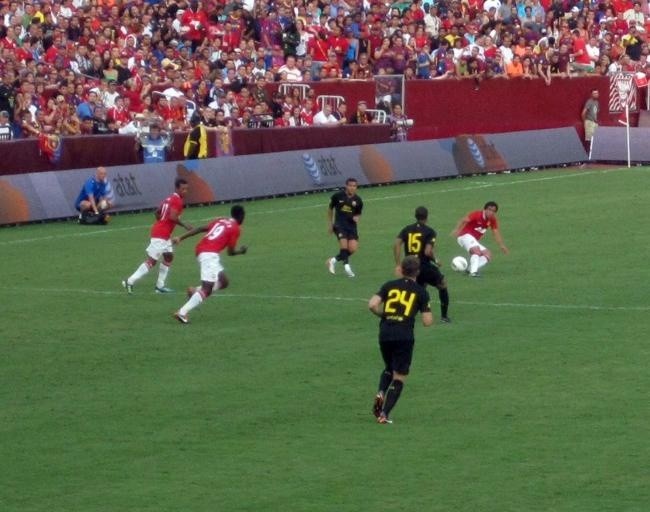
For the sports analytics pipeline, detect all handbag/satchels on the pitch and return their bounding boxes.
[82,209,108,225]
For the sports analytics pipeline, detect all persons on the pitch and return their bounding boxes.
[450,202,508,278]
[368,255,432,423]
[122,179,193,294]
[171,205,248,323]
[394,207,451,324]
[75,166,112,225]
[326,178,364,278]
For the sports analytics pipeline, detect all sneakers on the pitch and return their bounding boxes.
[171,309,190,324]
[346,270,355,278]
[466,269,479,278]
[372,392,385,417]
[122,279,133,295]
[376,417,393,424]
[440,318,451,324]
[325,256,336,274]
[187,284,194,297]
[154,286,175,294]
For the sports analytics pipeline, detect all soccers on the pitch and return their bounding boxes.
[451,256,468,272]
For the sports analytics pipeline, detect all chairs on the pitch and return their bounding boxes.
[0,125,16,140]
